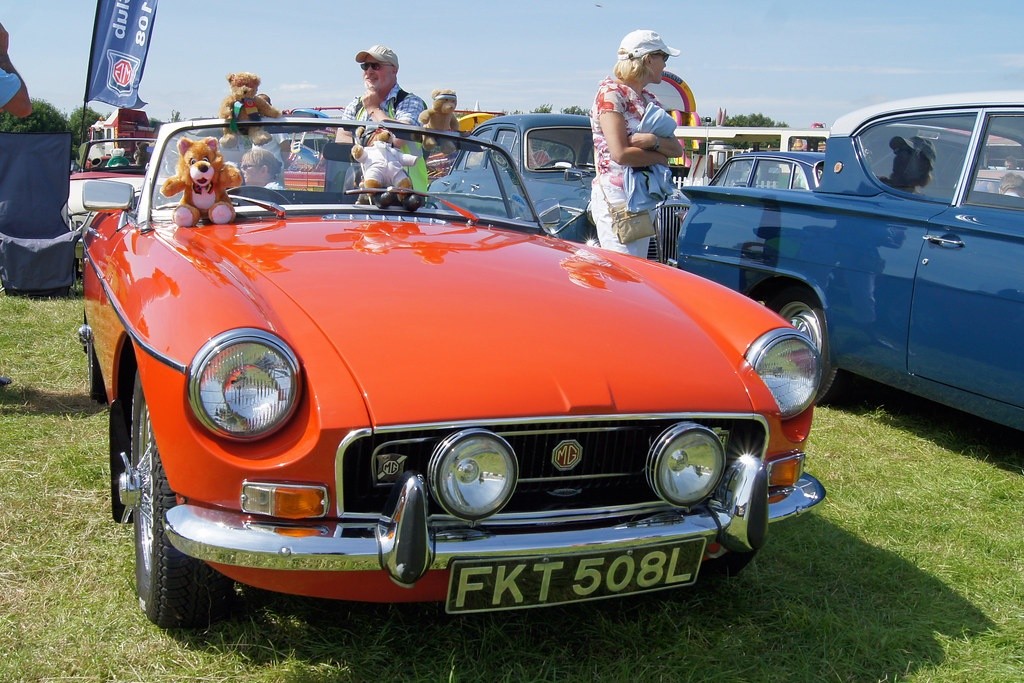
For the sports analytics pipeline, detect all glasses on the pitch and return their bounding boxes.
[240,164,258,172]
[893,146,915,155]
[360,63,394,70]
[650,52,669,62]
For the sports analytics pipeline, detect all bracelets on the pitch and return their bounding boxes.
[652,136,659,150]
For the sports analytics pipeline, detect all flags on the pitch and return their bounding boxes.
[86,0,157,109]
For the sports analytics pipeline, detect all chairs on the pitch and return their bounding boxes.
[0,130,92,296]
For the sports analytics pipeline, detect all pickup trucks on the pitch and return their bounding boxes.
[674,88,1024,438]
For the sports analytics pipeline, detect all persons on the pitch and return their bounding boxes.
[256,94,289,171]
[1005,156,1017,169]
[999,174,1024,198]
[878,136,936,193]
[335,45,426,144]
[0,24,32,117]
[241,148,283,189]
[589,30,681,258]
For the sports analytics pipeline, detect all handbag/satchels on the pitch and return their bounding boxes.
[610,201,655,244]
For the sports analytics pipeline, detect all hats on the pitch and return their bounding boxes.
[888,135,936,170]
[356,45,398,70]
[617,30,681,60]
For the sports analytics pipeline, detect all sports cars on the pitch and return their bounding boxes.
[73,116,827,631]
[66,136,187,239]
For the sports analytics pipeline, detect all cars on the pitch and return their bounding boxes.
[706,151,825,191]
[973,169,1024,198]
[425,111,692,269]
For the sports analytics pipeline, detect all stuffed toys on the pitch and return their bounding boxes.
[134,142,150,166]
[107,148,130,166]
[219,72,281,147]
[418,90,460,152]
[351,127,424,211]
[160,138,243,226]
